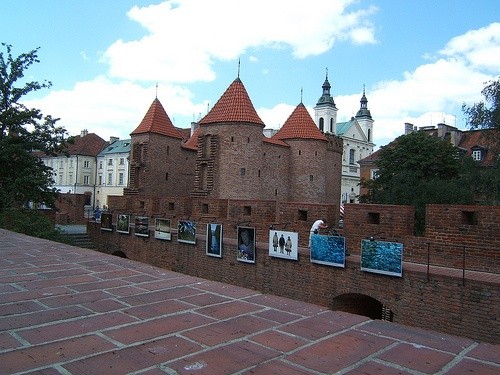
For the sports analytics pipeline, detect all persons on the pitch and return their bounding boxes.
[279,233,287,254]
[286,236,293,256]
[240,230,255,260]
[273,231,279,251]
[349,187,358,204]
[94,205,102,222]
[308,217,329,249]
